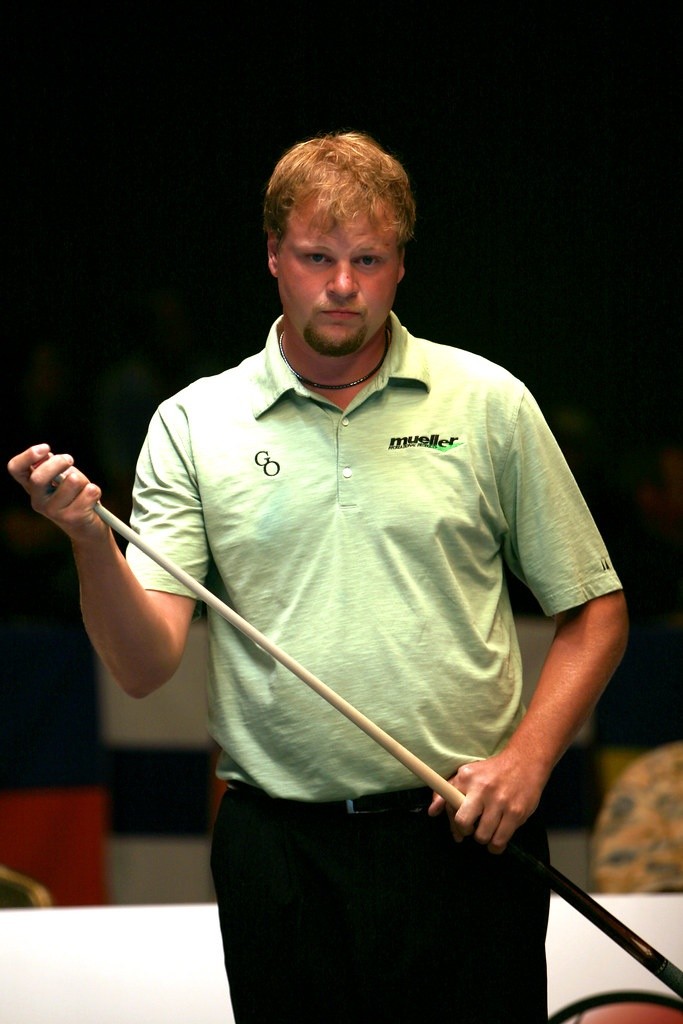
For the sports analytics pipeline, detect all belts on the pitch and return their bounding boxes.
[225,779,434,814]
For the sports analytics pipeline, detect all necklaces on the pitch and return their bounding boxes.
[279,323,389,389]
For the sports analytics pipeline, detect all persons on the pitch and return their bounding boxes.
[5,134,628,1024]
[0,266,224,625]
[500,396,683,628]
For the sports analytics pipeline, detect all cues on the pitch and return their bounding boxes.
[45,466,683,1001]
